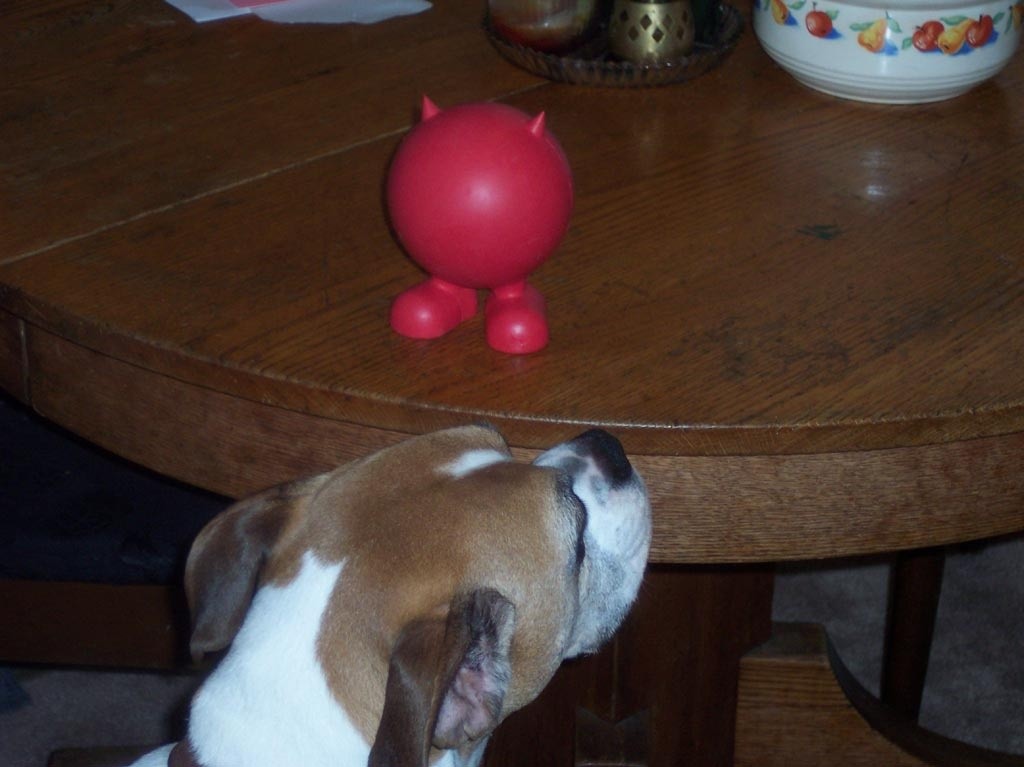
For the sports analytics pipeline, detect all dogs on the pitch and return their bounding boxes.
[127,424,651,765]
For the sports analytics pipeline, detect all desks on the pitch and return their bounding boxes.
[0,0,1024,766]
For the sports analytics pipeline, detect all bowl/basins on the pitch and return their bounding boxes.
[753,0,1023,105]
